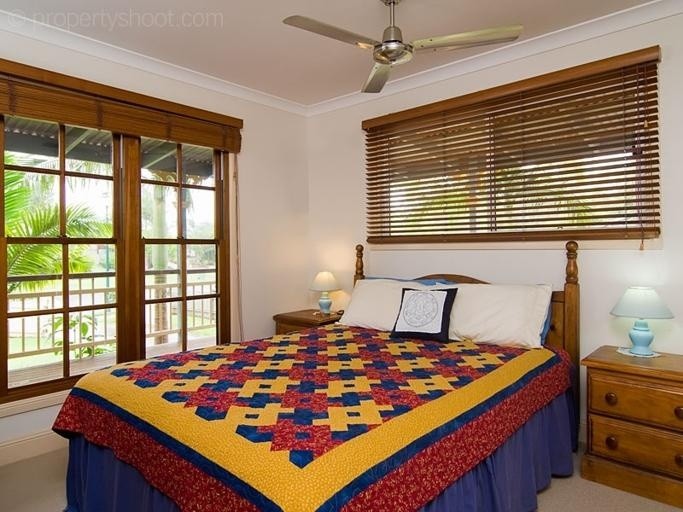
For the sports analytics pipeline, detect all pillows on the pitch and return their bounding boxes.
[336,276,554,349]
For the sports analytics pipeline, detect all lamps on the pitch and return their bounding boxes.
[610,287,675,356]
[310,271,342,315]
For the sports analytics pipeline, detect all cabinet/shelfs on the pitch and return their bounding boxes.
[579,344,683,510]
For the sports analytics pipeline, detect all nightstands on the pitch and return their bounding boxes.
[271,308,343,335]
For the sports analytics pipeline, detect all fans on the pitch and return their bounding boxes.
[282,0,524,92]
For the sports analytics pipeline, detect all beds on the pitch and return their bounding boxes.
[50,238,591,512]
